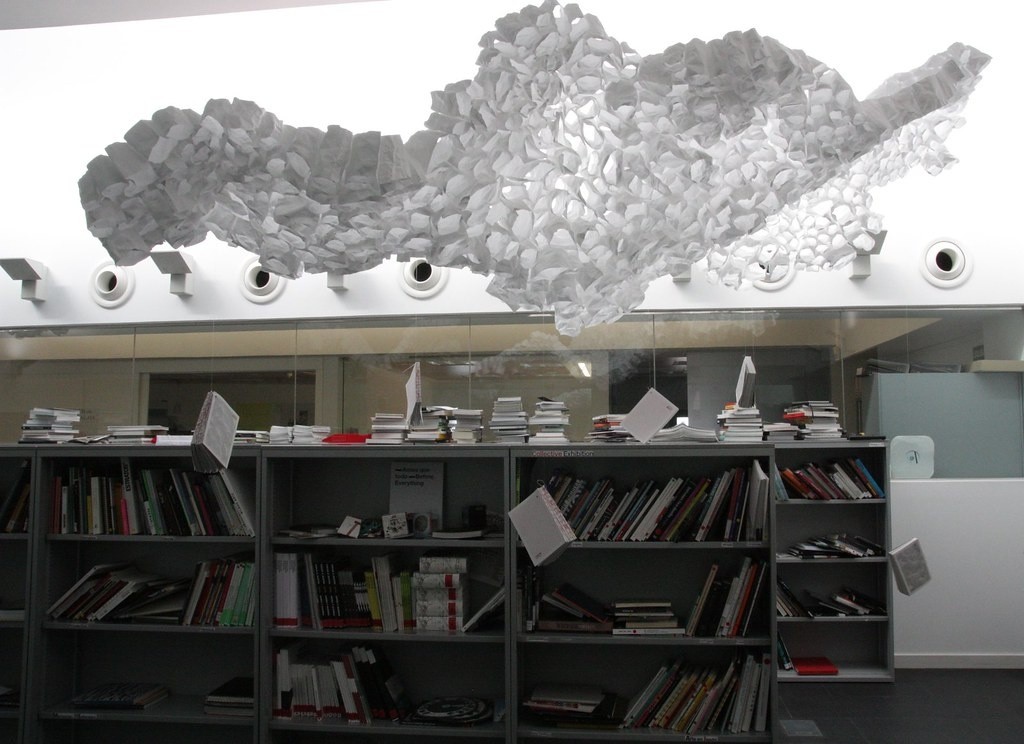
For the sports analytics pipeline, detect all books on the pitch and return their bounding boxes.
[584,412,633,444]
[403,362,424,427]
[15,408,81,444]
[619,386,678,444]
[0,538,888,744]
[278,462,506,541]
[526,400,573,445]
[642,399,799,441]
[777,530,886,562]
[71,397,484,442]
[734,355,757,408]
[488,396,532,442]
[509,486,575,568]
[890,537,934,595]
[525,459,767,542]
[189,390,240,474]
[0,457,30,532]
[785,399,850,441]
[775,457,884,500]
[47,457,256,538]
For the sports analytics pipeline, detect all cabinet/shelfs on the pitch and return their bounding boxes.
[0,372,1023,744]
